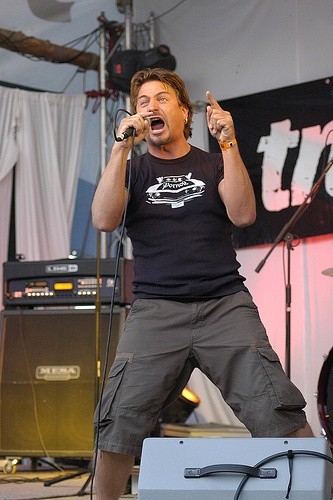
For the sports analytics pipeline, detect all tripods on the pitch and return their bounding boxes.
[44,230,103,496]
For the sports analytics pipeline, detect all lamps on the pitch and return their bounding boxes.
[162,387,200,424]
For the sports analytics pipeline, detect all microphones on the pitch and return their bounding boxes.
[117,126,136,141]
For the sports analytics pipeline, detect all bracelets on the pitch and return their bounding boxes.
[218,139,237,149]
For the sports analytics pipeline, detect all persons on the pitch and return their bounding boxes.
[90,69,314,500]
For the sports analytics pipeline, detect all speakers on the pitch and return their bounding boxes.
[0,306,130,460]
[137,438,330,500]
